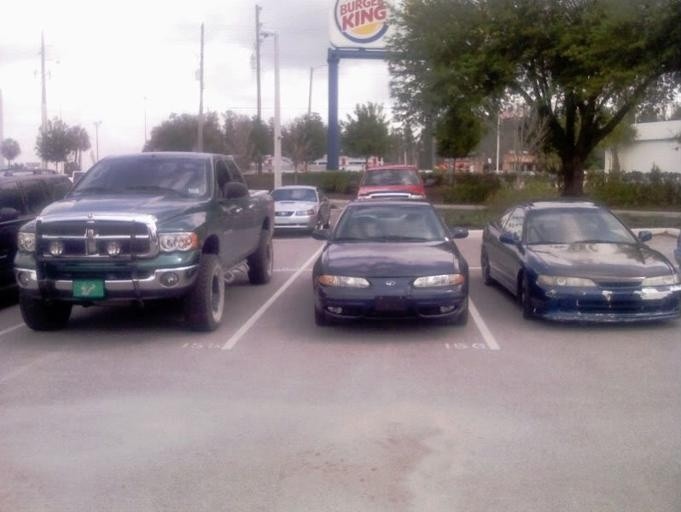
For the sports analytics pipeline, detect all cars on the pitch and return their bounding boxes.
[478,196,680,328]
[309,196,471,326]
[263,184,332,237]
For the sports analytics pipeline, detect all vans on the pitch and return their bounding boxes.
[355,163,439,206]
[309,160,327,172]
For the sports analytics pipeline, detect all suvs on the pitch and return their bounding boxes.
[0,165,72,305]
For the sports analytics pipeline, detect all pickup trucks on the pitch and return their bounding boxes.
[11,150,274,335]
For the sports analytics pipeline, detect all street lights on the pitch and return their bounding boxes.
[306,63,339,120]
